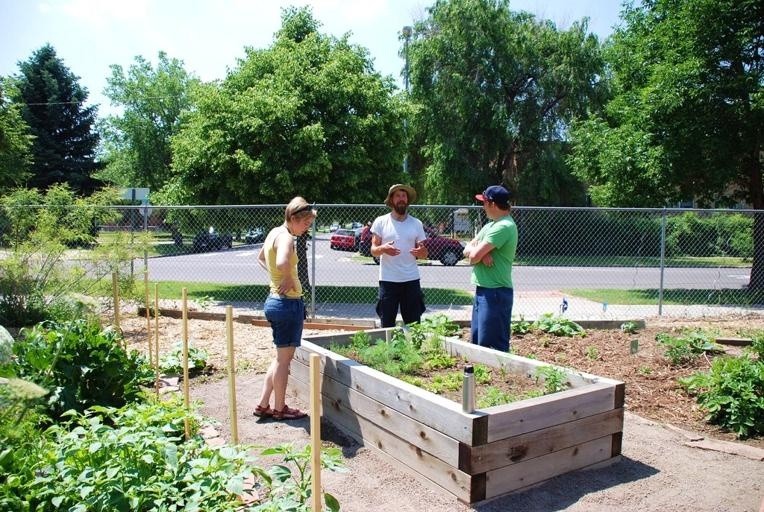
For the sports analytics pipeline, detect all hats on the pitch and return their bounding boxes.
[475,185,510,202]
[384,183,416,208]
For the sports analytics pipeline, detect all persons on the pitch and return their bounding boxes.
[252,195,318,421]
[369,182,430,328]
[461,184,520,354]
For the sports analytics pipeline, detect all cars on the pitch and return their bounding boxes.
[245,225,266,243]
[193,228,232,251]
[330,228,359,251]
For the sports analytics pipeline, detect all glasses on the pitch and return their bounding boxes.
[291,204,315,215]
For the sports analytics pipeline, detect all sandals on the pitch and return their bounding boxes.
[272,405,308,421]
[253,404,274,418]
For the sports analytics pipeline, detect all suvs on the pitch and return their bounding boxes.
[360,221,469,265]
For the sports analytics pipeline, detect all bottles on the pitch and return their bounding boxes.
[463,364,475,414]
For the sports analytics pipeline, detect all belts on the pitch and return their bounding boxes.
[269,293,302,300]
